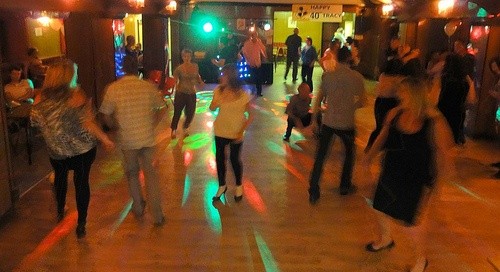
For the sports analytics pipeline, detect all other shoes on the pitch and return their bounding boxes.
[257,93,263,96]
[171,130,176,138]
[183,129,189,136]
[309,197,317,203]
[342,186,356,195]
[283,134,289,140]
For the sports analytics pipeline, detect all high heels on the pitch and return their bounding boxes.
[213,187,227,200]
[366,240,394,251]
[75,227,87,238]
[234,196,242,201]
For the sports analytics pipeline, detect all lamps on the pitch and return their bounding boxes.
[258,19,271,31]
[246,18,255,32]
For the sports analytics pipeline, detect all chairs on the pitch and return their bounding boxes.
[148,70,176,111]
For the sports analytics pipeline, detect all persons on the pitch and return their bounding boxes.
[237,31,269,97]
[170,49,206,138]
[27,46,50,74]
[208,65,255,203]
[300,35,317,94]
[3,65,37,118]
[210,33,240,68]
[99,55,169,229]
[116,35,143,79]
[311,27,360,113]
[362,36,499,171]
[284,27,302,83]
[305,47,372,203]
[361,75,454,272]
[283,83,323,141]
[30,58,115,240]
